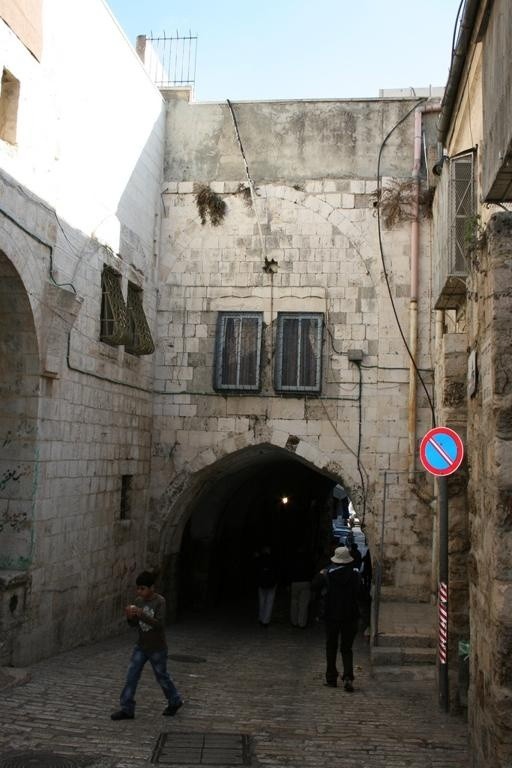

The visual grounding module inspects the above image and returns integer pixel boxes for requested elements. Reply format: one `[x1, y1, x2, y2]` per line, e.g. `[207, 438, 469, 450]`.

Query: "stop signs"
[419, 427, 464, 477]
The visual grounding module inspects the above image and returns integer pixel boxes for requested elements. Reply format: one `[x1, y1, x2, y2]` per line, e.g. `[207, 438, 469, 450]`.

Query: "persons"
[258, 543, 372, 630]
[111, 572, 182, 720]
[310, 546, 371, 692]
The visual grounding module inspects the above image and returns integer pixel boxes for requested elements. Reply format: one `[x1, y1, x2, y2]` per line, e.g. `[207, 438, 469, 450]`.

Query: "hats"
[331, 547, 354, 564]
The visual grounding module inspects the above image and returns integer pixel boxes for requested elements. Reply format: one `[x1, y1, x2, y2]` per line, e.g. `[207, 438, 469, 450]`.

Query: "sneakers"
[162, 700, 184, 717]
[343, 678, 353, 690]
[110, 711, 134, 720]
[323, 680, 336, 687]
